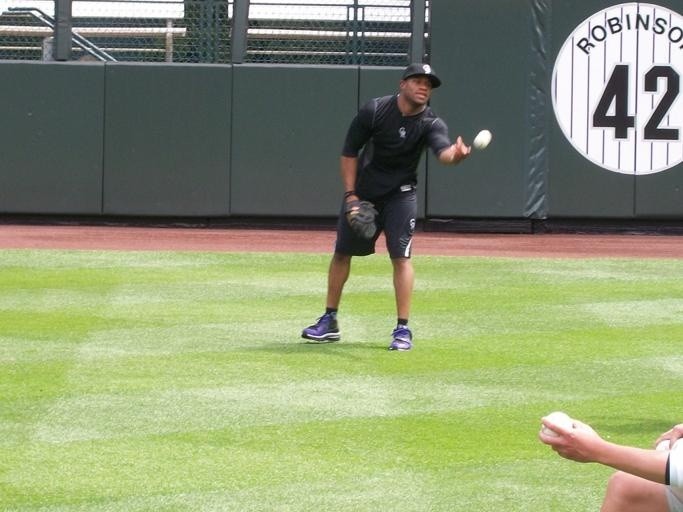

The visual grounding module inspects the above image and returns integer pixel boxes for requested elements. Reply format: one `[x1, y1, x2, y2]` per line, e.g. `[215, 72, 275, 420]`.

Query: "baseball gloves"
[344, 199, 380, 239]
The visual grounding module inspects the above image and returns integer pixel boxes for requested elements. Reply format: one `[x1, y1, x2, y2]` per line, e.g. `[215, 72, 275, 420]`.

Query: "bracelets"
[344, 191, 360, 200]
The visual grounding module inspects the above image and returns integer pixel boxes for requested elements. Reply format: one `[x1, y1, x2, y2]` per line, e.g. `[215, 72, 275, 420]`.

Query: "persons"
[298, 61, 471, 352]
[537, 410, 682, 512]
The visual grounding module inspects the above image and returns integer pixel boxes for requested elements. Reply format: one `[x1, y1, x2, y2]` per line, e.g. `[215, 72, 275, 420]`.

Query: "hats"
[403, 63, 441, 89]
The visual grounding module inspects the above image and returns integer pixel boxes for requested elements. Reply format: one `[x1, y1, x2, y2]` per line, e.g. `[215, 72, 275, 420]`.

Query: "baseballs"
[473, 129, 493, 149]
[541, 412, 572, 437]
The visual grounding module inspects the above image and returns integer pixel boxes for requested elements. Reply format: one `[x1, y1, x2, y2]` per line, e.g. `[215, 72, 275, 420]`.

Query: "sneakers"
[391, 324, 412, 351]
[300, 313, 339, 341]
